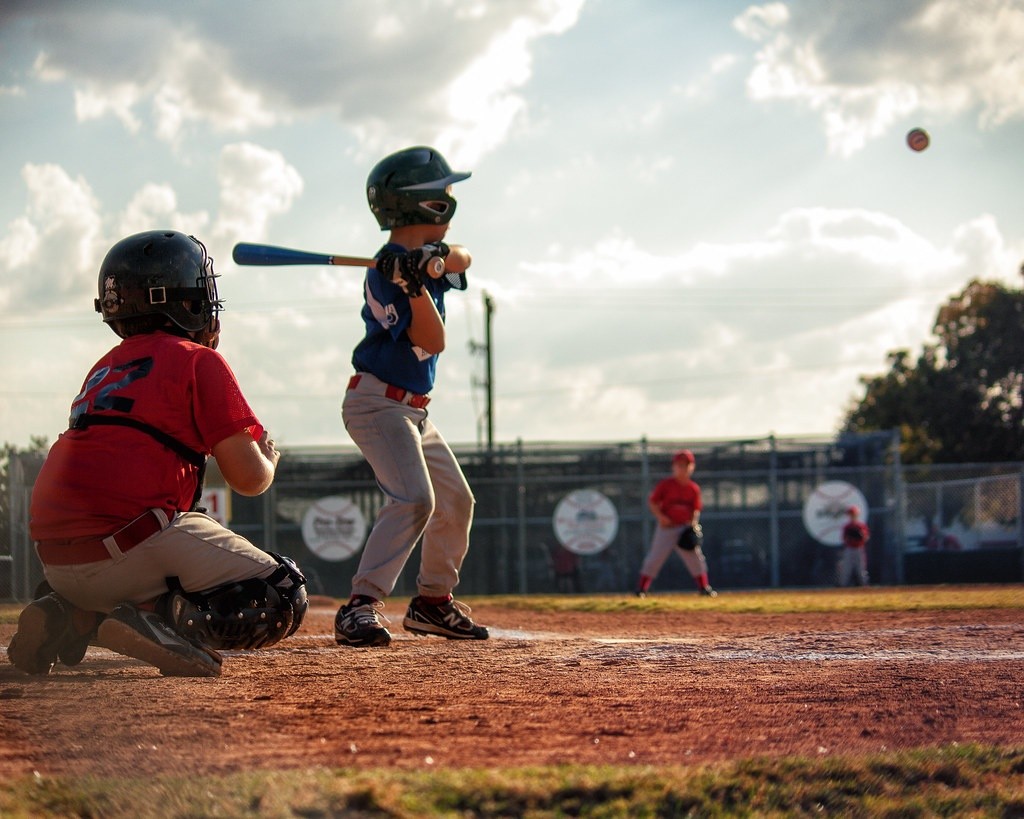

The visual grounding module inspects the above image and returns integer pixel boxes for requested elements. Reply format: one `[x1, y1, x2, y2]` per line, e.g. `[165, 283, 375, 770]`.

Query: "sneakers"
[334, 601, 394, 646]
[6, 592, 70, 675]
[401, 596, 489, 640]
[98, 603, 222, 678]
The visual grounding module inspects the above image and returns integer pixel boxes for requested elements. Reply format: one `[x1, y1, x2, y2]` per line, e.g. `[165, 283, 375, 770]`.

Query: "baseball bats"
[232, 242, 447, 279]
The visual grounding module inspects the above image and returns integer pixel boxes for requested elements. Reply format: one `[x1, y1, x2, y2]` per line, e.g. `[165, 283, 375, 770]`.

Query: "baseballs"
[906, 128, 931, 152]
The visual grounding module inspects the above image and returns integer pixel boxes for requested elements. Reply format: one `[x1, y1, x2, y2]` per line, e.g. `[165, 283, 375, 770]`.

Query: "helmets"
[93, 231, 225, 338]
[366, 145, 471, 230]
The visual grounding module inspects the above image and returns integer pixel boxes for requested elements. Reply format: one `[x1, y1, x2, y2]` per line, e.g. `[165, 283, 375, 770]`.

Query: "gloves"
[377, 252, 426, 297]
[402, 241, 451, 274]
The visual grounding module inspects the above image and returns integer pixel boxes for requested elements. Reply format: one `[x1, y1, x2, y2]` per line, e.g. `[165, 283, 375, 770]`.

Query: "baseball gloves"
[843, 527, 864, 540]
[677, 525, 704, 551]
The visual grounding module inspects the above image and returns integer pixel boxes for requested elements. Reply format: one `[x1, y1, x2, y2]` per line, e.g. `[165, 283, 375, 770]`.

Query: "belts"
[346, 376, 431, 408]
[38, 508, 175, 565]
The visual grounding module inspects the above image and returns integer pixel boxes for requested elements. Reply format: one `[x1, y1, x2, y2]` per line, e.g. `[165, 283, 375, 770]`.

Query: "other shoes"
[700, 585, 718, 597]
[636, 591, 646, 598]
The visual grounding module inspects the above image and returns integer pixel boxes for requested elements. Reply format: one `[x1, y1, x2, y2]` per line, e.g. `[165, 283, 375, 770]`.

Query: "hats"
[672, 450, 695, 463]
[848, 507, 860, 515]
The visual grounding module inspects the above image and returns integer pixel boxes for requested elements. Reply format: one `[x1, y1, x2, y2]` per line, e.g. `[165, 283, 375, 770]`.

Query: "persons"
[8, 230, 309, 679]
[636, 449, 715, 598]
[837, 506, 870, 587]
[335, 145, 490, 646]
